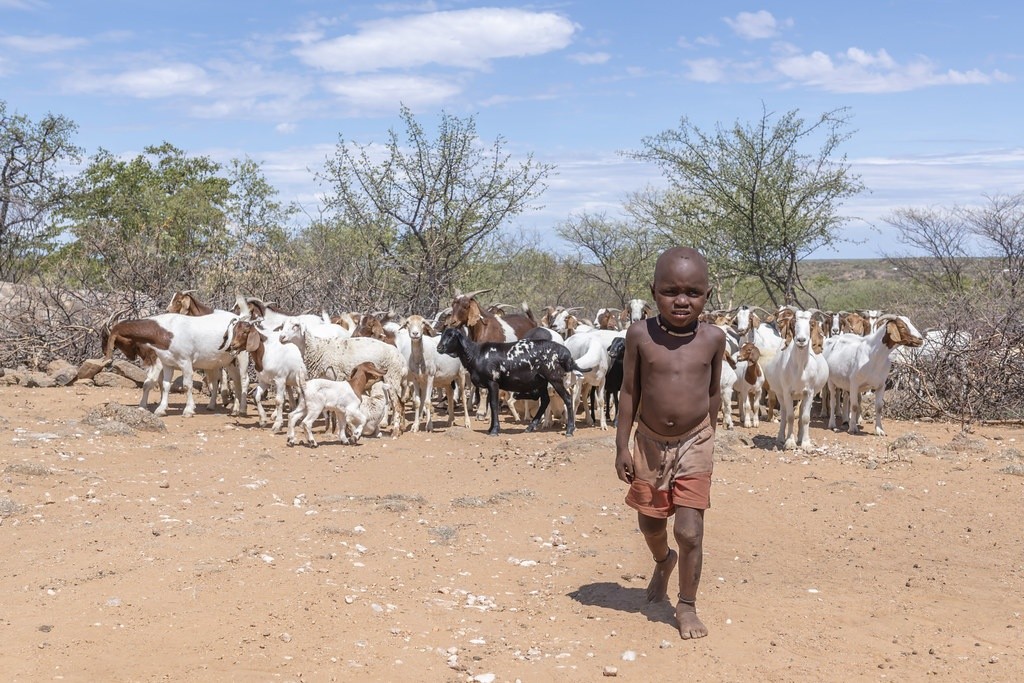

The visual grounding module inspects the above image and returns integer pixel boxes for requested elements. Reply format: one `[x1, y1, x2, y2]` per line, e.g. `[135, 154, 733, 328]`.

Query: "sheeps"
[96, 276, 975, 455]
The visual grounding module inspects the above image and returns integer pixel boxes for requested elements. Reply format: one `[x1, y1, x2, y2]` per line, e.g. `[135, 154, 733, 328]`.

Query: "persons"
[615, 246, 727, 640]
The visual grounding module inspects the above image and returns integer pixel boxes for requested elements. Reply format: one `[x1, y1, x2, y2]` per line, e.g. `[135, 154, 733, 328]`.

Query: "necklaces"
[656, 314, 700, 337]
